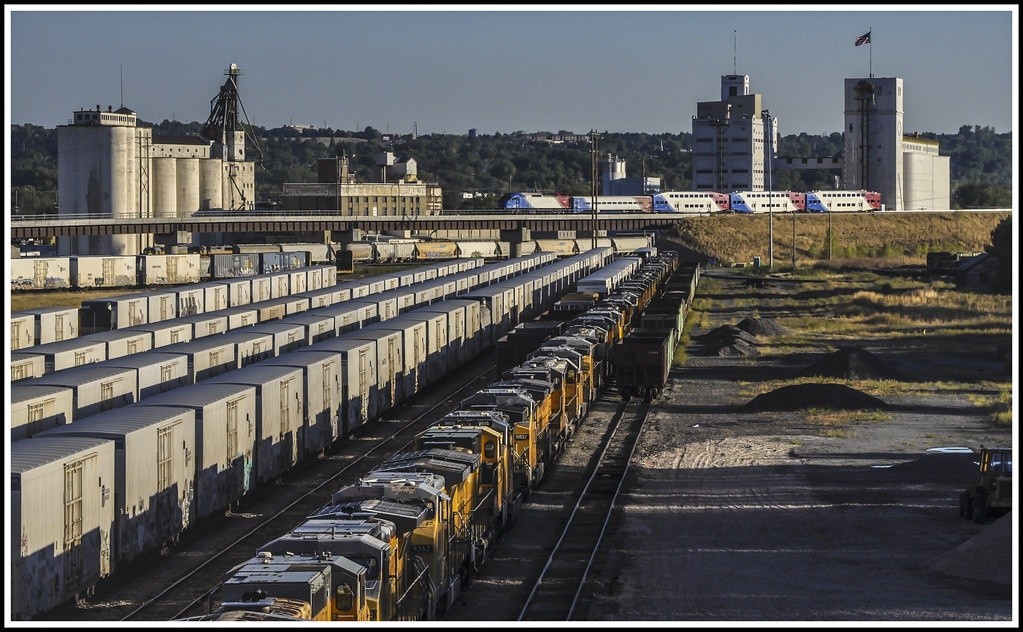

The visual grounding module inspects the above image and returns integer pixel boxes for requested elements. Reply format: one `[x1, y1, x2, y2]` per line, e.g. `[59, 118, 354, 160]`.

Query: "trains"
[497, 188, 882, 214]
[208, 246, 679, 622]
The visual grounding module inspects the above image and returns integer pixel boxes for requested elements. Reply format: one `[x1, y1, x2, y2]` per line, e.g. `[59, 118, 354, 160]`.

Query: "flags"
[855, 31, 870, 47]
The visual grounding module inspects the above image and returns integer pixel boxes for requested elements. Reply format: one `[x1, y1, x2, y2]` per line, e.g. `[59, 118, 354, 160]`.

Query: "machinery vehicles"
[960, 444, 1012, 524]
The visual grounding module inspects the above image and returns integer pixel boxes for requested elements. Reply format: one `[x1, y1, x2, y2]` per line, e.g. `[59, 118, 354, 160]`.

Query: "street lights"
[761, 110, 774, 269]
